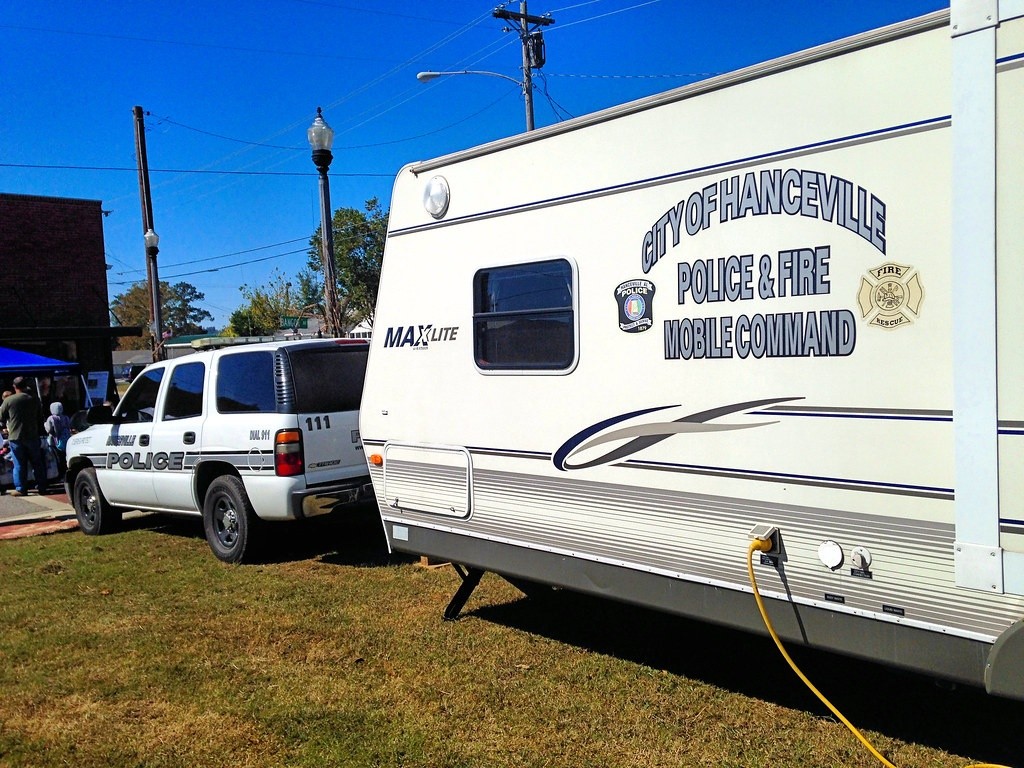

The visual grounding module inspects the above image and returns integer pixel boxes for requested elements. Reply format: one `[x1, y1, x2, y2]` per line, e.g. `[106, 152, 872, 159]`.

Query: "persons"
[0, 376, 54, 496]
[44, 402, 71, 482]
[86, 394, 120, 425]
[0, 391, 12, 404]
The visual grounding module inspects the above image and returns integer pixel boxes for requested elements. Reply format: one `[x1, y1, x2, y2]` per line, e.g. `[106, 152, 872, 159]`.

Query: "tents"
[1, 346, 96, 487]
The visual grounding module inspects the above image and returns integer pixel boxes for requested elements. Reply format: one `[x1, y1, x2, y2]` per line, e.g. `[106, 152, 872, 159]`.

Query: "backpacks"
[51, 416, 71, 451]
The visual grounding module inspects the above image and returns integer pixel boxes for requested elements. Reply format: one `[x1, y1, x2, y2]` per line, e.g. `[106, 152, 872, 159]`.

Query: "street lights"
[143, 227, 167, 361]
[415, 68, 536, 132]
[307, 108, 338, 334]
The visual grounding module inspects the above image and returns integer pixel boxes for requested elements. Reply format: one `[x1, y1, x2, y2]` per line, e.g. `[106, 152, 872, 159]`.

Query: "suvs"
[121, 365, 143, 382]
[63, 337, 374, 565]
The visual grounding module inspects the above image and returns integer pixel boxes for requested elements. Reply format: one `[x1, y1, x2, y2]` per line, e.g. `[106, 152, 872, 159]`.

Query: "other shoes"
[11, 490, 26, 496]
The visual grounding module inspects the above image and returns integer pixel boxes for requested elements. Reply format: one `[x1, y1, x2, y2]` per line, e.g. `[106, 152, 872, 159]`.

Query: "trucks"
[359, 4, 1024, 716]
